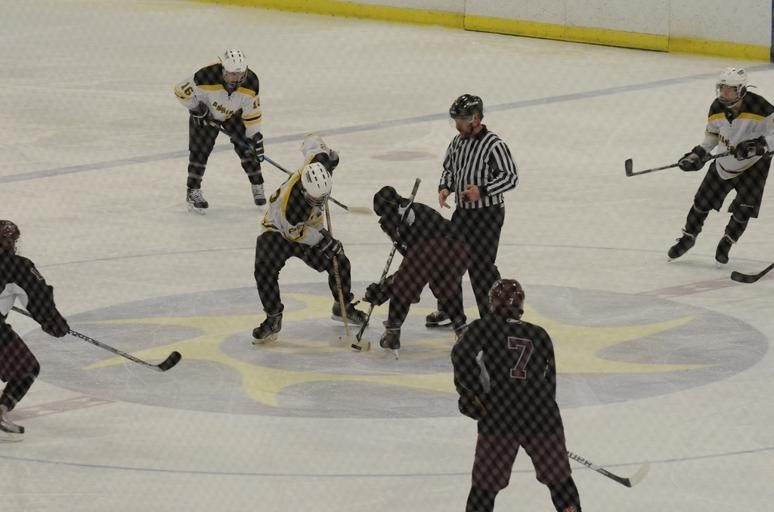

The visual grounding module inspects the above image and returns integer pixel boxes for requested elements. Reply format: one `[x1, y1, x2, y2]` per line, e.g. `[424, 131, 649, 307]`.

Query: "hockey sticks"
[624, 151, 732, 176]
[354, 179, 422, 350]
[10, 306, 181, 372]
[565, 452, 650, 487]
[209, 122, 373, 216]
[327, 201, 355, 348]
[730, 261, 774, 283]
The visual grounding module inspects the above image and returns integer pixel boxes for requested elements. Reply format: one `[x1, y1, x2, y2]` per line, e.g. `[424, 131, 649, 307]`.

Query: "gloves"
[458, 395, 482, 419]
[40, 315, 71, 338]
[190, 101, 213, 128]
[733, 134, 765, 161]
[364, 282, 389, 307]
[679, 145, 709, 171]
[245, 131, 265, 162]
[317, 228, 344, 261]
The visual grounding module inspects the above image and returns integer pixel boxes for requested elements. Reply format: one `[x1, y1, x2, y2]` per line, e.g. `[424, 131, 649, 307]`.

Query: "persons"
[421, 94, 521, 321]
[0, 220, 69, 434]
[249, 131, 369, 341]
[450, 277, 585, 512]
[361, 184, 473, 352]
[666, 68, 772, 263]
[173, 48, 269, 208]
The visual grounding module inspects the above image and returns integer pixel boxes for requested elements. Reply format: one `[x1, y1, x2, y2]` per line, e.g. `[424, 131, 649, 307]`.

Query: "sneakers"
[426, 309, 451, 324]
[251, 182, 267, 206]
[187, 186, 209, 208]
[715, 234, 736, 264]
[380, 327, 402, 349]
[668, 228, 696, 258]
[332, 299, 369, 323]
[0, 405, 26, 434]
[253, 312, 282, 339]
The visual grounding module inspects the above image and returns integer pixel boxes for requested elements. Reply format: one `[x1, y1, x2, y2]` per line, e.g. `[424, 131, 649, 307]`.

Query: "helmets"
[0, 220, 21, 240]
[450, 94, 484, 118]
[717, 67, 748, 98]
[488, 278, 524, 313]
[302, 162, 333, 198]
[221, 48, 249, 73]
